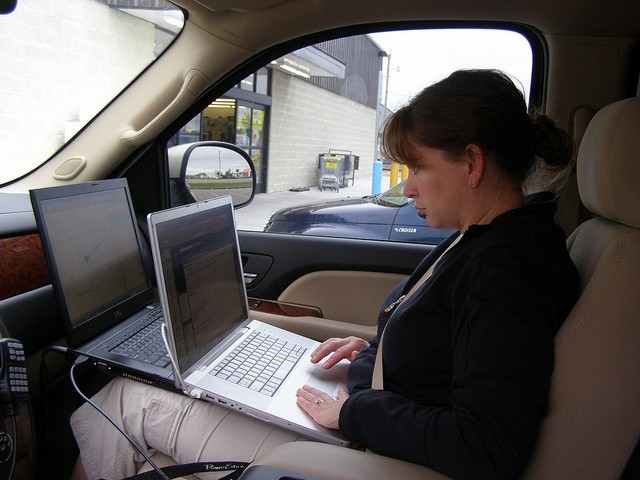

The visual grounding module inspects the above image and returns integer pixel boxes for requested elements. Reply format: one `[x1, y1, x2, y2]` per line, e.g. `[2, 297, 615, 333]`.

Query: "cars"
[260, 166, 458, 246]
[0, 0, 631, 480]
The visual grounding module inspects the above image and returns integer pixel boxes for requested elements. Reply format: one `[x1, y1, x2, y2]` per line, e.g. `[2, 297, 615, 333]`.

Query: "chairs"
[136, 97, 640, 480]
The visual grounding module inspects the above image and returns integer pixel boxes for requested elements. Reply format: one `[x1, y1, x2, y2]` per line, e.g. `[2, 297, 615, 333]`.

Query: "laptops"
[29, 177, 182, 393]
[147, 195, 351, 448]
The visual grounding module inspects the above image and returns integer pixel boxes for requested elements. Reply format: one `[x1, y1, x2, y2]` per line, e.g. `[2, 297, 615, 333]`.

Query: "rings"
[315, 399, 326, 406]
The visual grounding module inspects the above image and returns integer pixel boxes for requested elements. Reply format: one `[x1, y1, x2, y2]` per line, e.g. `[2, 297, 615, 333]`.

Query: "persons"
[69, 68, 586, 478]
